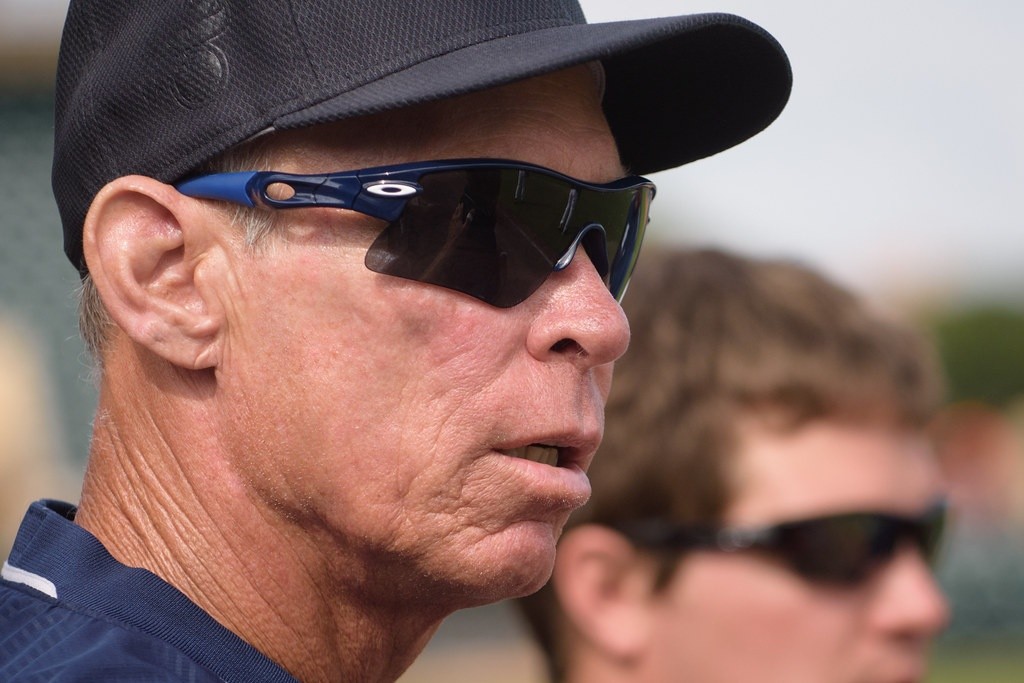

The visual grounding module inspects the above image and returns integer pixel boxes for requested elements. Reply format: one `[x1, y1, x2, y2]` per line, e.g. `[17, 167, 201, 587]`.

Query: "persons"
[505, 241, 952, 683]
[1, 1, 792, 683]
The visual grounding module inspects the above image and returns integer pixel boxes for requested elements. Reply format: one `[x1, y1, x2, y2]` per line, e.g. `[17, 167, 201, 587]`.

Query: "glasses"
[618, 498, 949, 587]
[174, 157, 657, 310]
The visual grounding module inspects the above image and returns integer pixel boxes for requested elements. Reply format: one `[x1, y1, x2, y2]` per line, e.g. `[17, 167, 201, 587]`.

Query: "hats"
[53, 1, 793, 281]
